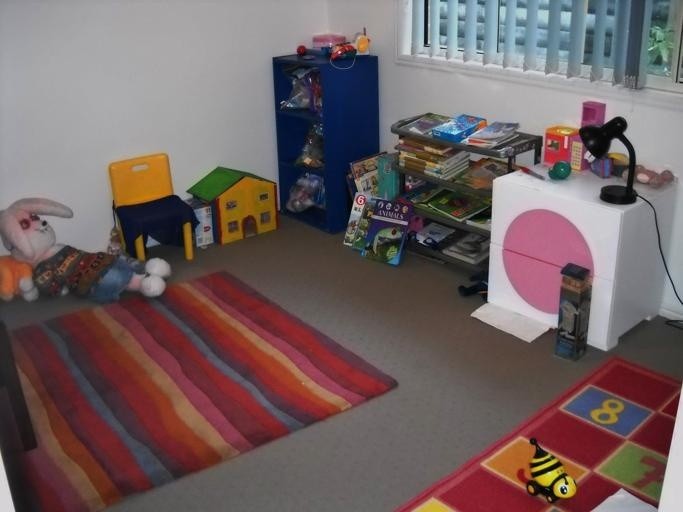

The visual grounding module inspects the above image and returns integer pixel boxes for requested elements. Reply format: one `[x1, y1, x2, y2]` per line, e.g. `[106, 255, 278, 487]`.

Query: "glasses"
[579, 116, 637, 204]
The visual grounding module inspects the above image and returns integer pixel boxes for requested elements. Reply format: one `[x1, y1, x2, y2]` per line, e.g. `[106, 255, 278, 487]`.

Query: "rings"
[519, 166, 545, 181]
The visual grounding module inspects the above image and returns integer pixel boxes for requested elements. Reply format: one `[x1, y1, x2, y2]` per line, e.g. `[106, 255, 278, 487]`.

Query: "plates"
[388, 113, 542, 281]
[486, 157, 678, 351]
[271, 54, 378, 232]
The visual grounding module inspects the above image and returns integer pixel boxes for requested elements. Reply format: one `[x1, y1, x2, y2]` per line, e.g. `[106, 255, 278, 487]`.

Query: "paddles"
[396, 358, 681, 512]
[10, 270, 398, 512]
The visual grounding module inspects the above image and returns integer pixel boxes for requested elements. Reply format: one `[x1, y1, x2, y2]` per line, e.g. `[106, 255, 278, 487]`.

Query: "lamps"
[109, 153, 194, 263]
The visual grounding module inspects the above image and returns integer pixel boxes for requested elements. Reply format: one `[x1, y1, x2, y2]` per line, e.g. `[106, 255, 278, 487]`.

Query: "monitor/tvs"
[343, 112, 520, 265]
